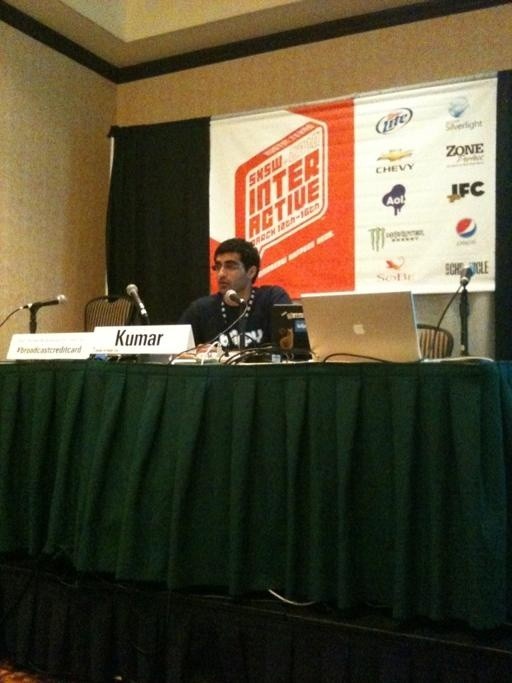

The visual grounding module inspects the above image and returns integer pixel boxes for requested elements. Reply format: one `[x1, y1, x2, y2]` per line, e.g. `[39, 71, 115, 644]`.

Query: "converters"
[242, 350, 273, 363]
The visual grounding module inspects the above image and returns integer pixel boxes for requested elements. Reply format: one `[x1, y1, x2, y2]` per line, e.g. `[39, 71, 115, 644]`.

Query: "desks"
[0, 357, 511, 629]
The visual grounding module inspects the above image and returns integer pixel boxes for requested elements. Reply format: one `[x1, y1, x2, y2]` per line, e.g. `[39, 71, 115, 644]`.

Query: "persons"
[178, 238, 293, 354]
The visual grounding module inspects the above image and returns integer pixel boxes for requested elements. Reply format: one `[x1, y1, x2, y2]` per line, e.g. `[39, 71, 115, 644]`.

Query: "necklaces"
[221, 289, 255, 324]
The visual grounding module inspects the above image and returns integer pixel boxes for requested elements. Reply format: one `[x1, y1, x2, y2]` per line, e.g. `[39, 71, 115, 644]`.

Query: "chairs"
[84, 295, 134, 332]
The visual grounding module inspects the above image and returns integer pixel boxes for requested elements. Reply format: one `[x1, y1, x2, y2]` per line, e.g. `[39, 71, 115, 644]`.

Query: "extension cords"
[196, 348, 270, 361]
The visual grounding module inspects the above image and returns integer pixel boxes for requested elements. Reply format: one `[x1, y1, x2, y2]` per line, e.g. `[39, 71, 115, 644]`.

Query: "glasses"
[211, 262, 248, 271]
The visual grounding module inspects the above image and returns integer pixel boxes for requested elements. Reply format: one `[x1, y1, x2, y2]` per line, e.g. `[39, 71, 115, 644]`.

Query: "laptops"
[270, 303, 312, 361]
[301, 290, 423, 362]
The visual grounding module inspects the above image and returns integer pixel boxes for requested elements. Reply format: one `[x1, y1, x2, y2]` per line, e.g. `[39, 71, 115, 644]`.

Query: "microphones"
[21, 294, 67, 311]
[126, 285, 149, 318]
[460, 262, 476, 285]
[225, 289, 245, 306]
